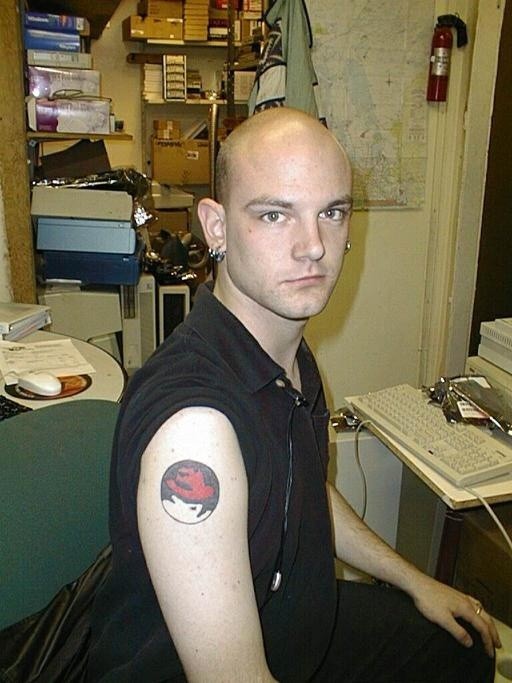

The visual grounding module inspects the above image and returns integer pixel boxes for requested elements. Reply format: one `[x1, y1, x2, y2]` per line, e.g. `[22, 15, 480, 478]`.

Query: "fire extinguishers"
[427, 14, 468, 101]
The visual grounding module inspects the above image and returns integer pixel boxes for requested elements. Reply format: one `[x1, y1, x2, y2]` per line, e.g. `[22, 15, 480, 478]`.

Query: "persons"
[104, 103, 502, 682]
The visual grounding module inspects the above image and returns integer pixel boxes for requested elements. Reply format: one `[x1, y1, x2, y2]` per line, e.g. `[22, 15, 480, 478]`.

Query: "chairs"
[0, 398, 131, 628]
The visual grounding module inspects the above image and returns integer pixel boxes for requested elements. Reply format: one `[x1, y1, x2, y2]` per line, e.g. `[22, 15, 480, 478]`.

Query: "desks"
[2, 328, 127, 412]
[347, 375, 512, 511]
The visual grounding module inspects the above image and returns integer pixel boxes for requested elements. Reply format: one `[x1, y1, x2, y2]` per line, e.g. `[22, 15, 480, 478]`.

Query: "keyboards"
[0, 395, 32, 424]
[351, 383, 512, 489]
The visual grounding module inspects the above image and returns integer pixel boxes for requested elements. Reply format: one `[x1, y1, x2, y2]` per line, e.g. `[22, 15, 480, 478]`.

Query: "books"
[179, 1, 264, 43]
[182, 119, 207, 140]
[1, 298, 53, 341]
[140, 61, 165, 103]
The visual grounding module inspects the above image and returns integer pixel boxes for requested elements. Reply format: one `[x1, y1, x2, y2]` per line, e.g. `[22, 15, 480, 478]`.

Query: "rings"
[474, 606, 482, 615]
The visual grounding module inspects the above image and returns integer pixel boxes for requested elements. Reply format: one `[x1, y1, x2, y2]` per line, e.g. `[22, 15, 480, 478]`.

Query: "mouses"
[18, 372, 62, 396]
